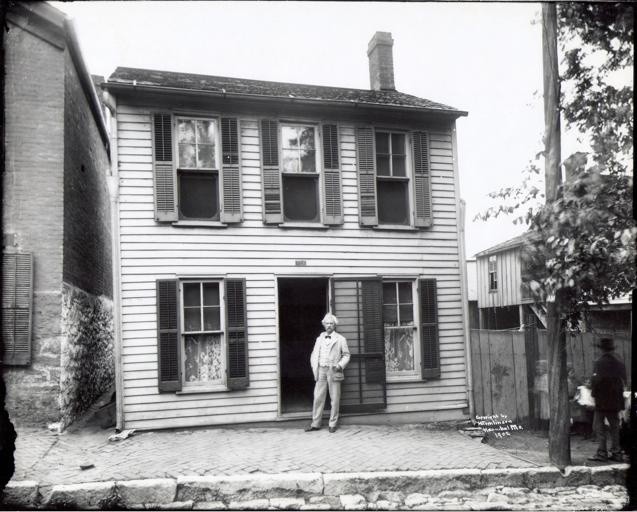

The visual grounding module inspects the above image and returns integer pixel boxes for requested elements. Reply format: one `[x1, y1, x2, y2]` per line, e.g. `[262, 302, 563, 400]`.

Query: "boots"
[609, 453, 622, 462]
[587, 450, 608, 462]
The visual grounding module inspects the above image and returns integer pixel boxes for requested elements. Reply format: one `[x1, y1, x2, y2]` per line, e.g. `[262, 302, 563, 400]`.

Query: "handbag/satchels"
[333, 372, 344, 381]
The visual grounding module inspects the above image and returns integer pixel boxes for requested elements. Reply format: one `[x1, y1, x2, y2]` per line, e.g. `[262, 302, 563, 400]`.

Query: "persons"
[585, 335, 628, 464]
[532, 359, 550, 439]
[566, 364, 581, 434]
[569, 375, 595, 441]
[304, 313, 351, 434]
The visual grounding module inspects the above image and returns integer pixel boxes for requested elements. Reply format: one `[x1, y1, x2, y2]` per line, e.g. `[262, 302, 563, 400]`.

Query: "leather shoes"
[305, 427, 320, 431]
[329, 427, 336, 433]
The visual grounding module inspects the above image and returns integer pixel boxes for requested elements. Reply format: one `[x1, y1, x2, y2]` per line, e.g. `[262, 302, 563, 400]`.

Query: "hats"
[594, 338, 615, 350]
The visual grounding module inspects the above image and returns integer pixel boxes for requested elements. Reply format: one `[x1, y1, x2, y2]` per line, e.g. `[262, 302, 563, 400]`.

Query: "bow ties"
[325, 336, 331, 339]
